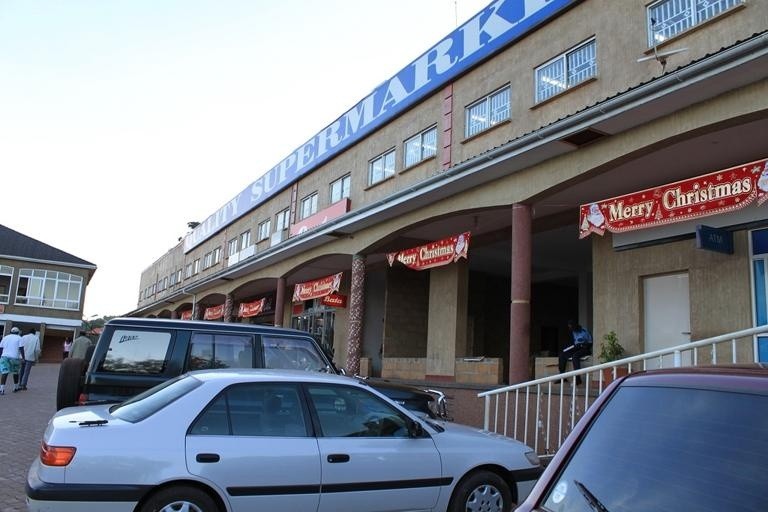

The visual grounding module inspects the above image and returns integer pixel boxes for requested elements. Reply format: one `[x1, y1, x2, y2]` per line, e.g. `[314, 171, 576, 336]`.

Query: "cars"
[25, 369, 543, 512]
[511, 362, 766, 512]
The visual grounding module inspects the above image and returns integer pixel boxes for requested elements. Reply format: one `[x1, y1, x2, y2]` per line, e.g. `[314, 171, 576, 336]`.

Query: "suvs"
[56, 317, 452, 423]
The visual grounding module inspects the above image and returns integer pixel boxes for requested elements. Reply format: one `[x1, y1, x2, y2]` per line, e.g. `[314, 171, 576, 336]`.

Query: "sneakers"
[13, 386, 27, 392]
[0, 390, 4, 395]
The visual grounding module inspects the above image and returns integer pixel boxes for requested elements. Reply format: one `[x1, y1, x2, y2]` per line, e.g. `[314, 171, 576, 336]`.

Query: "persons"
[18, 328, 41, 390]
[63, 336, 72, 360]
[0, 327, 25, 394]
[554, 320, 592, 385]
[69, 329, 92, 358]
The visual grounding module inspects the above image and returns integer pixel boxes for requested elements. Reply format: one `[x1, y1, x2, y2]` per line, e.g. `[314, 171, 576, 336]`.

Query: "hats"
[11, 327, 19, 333]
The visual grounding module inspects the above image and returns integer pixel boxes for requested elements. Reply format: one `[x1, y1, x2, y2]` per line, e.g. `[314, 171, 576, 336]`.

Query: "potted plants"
[598, 331, 632, 388]
